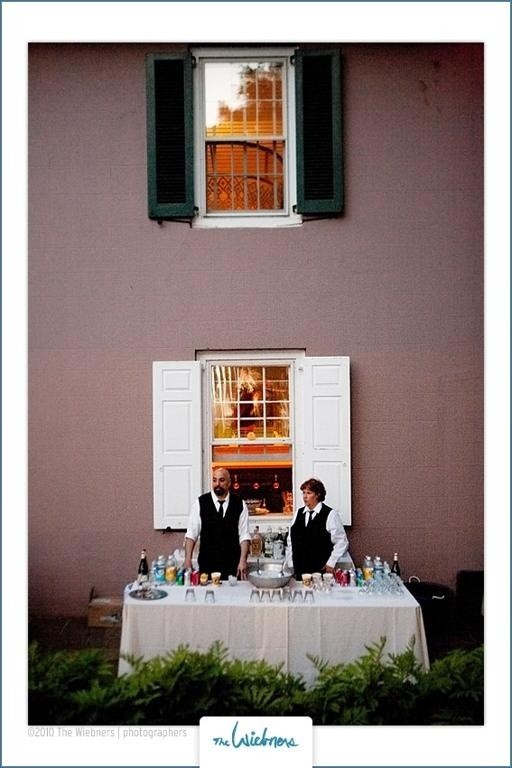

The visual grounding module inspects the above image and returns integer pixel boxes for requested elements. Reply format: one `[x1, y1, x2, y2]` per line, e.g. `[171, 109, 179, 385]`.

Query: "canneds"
[335, 568, 383, 588]
[191, 572, 200, 586]
[176, 571, 184, 586]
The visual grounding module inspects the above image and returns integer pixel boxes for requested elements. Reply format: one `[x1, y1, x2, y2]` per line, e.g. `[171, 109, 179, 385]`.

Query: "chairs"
[456, 568, 483, 613]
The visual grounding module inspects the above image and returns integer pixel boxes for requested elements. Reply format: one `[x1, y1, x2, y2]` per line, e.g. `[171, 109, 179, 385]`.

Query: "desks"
[115, 577, 427, 687]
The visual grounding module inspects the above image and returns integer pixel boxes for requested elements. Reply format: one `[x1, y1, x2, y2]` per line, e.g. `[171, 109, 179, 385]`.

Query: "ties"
[307, 510, 314, 527]
[218, 500, 225, 518]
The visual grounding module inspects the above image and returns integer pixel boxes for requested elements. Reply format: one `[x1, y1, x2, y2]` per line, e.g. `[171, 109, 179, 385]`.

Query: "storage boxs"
[88, 601, 125, 629]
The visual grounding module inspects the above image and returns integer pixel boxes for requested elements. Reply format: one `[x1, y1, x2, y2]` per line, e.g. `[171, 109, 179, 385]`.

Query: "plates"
[128, 588, 168, 600]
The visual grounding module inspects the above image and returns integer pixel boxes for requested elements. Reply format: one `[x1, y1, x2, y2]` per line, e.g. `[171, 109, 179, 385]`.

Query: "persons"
[282, 478, 349, 582]
[181, 468, 251, 582]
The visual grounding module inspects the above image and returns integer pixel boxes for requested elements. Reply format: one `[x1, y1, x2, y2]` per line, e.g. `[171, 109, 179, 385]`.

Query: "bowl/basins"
[244, 498, 261, 512]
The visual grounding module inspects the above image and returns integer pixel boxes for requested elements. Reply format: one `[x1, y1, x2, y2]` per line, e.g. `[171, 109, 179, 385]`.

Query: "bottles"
[362, 552, 401, 580]
[335, 567, 363, 586]
[138, 549, 149, 584]
[250, 524, 290, 559]
[152, 554, 209, 586]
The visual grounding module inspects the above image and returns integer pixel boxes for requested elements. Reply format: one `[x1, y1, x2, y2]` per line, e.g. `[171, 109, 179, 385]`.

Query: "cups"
[302, 571, 334, 598]
[228, 574, 237, 585]
[185, 588, 196, 602]
[137, 568, 161, 597]
[211, 571, 222, 585]
[248, 588, 314, 604]
[330, 586, 355, 600]
[283, 490, 292, 513]
[359, 571, 405, 603]
[204, 590, 216, 603]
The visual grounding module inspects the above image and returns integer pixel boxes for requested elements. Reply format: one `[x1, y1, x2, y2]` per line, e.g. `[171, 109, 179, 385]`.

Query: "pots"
[240, 564, 293, 588]
